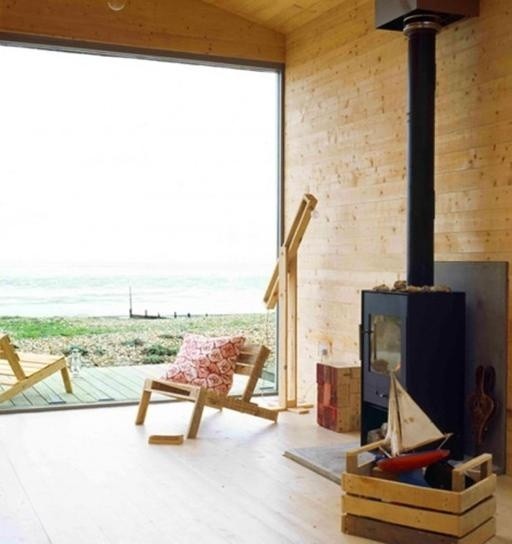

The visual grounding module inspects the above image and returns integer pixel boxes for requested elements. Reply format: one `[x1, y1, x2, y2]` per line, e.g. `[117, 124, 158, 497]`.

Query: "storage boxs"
[341, 440, 495, 544]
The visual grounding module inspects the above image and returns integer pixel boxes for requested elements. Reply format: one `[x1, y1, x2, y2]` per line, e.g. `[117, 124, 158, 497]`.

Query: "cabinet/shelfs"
[317, 361, 361, 433]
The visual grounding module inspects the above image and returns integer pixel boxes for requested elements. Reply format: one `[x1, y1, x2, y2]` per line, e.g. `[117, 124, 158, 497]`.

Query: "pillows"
[165, 332, 245, 395]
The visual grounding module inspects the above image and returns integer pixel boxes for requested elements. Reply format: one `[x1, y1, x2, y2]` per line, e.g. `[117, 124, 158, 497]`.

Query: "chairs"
[0, 331, 281, 437]
